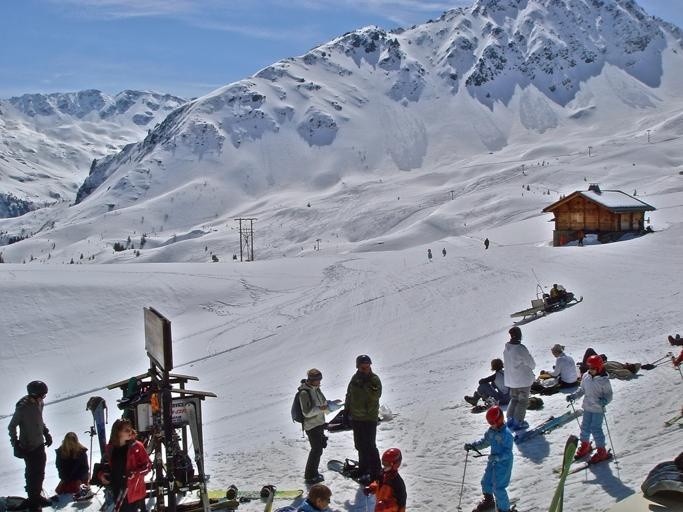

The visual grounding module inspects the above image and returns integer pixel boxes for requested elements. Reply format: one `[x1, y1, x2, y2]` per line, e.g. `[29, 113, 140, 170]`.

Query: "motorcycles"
[509, 281, 583, 325]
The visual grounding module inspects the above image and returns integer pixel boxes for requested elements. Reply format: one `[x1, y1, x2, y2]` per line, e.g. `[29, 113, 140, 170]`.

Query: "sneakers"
[476, 497, 493, 511]
[675, 334, 680, 339]
[513, 423, 528, 430]
[667, 336, 674, 346]
[506, 417, 513, 428]
[590, 448, 607, 463]
[464, 396, 477, 406]
[573, 442, 590, 458]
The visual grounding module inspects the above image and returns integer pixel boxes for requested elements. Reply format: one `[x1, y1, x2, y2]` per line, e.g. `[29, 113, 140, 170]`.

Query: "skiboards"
[665, 409, 683, 428]
[547, 436, 579, 512]
[470, 497, 539, 512]
[513, 409, 583, 445]
[552, 444, 630, 478]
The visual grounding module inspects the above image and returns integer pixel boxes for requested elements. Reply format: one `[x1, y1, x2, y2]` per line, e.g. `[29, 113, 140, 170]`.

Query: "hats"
[308, 369, 321, 381]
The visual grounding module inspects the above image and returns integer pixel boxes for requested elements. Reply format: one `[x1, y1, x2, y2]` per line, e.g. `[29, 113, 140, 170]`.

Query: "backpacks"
[290, 389, 312, 439]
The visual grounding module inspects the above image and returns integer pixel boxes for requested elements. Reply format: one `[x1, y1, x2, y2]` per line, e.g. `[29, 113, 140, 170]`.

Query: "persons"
[484, 238, 490, 250]
[566, 355, 612, 464]
[292, 367, 342, 483]
[540, 344, 578, 388]
[464, 359, 510, 407]
[54, 430, 95, 501]
[503, 326, 536, 430]
[464, 406, 518, 512]
[8, 381, 54, 512]
[364, 447, 408, 512]
[550, 284, 563, 300]
[344, 354, 382, 482]
[293, 485, 332, 512]
[96, 418, 153, 512]
[578, 229, 584, 247]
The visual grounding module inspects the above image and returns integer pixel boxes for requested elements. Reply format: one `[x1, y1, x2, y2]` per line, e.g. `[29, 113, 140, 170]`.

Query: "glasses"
[381, 461, 389, 466]
[586, 367, 594, 370]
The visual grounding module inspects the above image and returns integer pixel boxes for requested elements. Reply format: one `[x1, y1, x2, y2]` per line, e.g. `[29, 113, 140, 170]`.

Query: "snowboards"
[196, 488, 303, 500]
[326, 460, 386, 487]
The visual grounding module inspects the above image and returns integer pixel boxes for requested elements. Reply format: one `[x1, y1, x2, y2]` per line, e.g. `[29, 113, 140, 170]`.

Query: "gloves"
[463, 444, 470, 450]
[363, 486, 371, 496]
[45, 434, 51, 447]
[598, 399, 607, 406]
[672, 358, 680, 366]
[487, 455, 499, 464]
[565, 395, 570, 402]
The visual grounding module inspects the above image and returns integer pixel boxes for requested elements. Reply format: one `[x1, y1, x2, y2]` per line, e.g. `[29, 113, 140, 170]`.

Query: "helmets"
[381, 449, 402, 468]
[356, 355, 371, 364]
[491, 358, 502, 370]
[585, 355, 603, 373]
[485, 406, 503, 427]
[27, 381, 47, 398]
